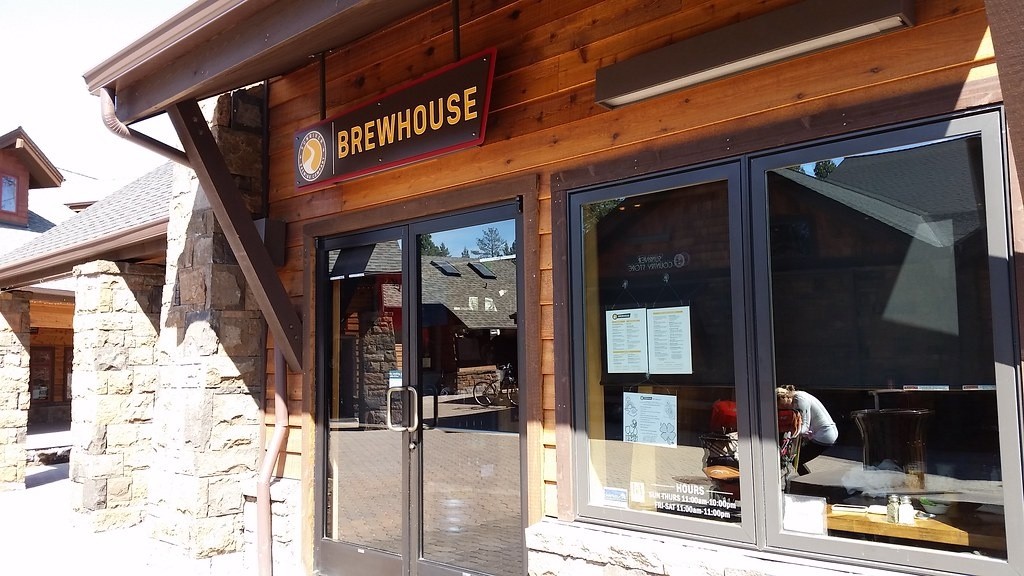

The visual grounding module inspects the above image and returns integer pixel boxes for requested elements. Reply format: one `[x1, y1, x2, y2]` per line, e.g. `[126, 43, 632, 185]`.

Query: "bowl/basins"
[921, 503, 952, 514]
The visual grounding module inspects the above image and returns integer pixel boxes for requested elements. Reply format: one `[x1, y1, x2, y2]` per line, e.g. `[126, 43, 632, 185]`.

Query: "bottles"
[898, 494, 914, 525]
[887, 494, 899, 524]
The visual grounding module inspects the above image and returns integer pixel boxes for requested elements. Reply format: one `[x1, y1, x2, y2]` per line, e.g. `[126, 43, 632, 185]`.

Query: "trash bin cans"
[849, 408, 930, 473]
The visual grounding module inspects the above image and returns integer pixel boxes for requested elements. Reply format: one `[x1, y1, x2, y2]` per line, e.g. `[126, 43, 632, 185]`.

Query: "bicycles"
[472, 363, 519, 408]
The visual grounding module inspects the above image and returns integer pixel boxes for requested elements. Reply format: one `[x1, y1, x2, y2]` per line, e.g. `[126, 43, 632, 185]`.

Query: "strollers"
[778, 409, 802, 474]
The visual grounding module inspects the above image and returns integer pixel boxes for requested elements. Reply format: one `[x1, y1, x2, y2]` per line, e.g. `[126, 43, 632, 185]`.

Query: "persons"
[777, 386, 838, 477]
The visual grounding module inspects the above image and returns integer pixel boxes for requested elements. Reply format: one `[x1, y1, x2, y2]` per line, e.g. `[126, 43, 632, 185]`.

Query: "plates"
[867, 505, 887, 515]
